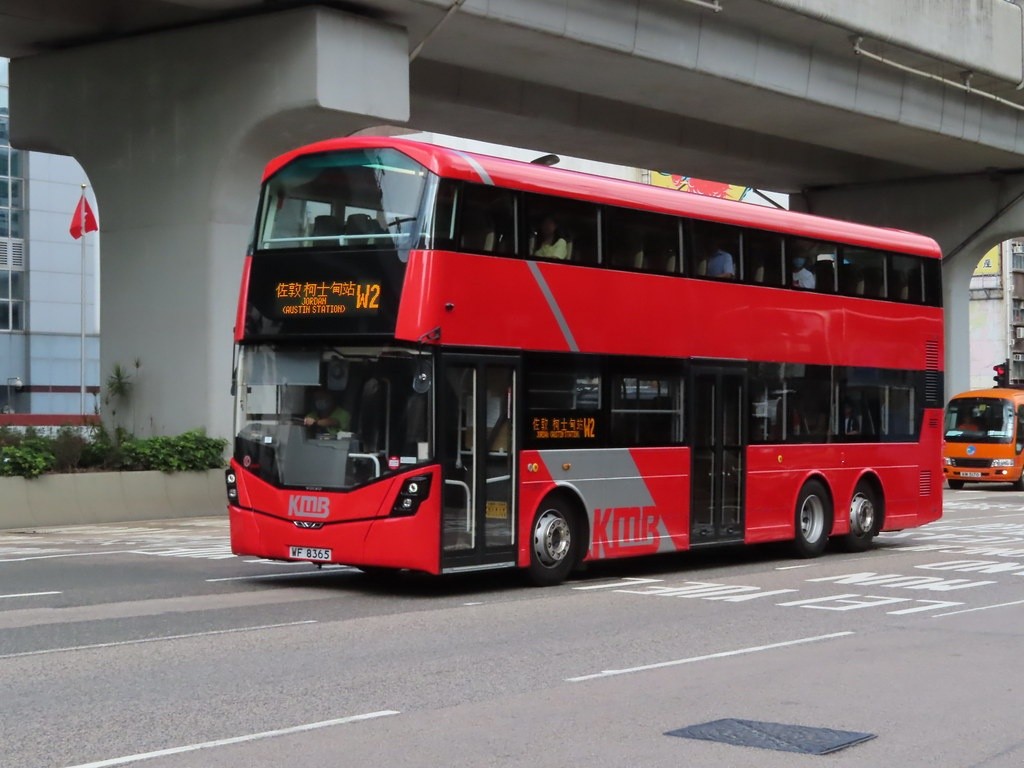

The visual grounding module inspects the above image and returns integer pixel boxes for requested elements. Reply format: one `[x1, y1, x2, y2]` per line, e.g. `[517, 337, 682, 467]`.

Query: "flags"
[69, 196, 98, 239]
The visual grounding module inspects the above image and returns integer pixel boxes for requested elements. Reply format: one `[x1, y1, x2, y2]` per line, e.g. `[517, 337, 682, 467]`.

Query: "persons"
[960, 415, 977, 431]
[304, 391, 351, 435]
[534, 215, 568, 258]
[786, 251, 815, 288]
[701, 239, 733, 279]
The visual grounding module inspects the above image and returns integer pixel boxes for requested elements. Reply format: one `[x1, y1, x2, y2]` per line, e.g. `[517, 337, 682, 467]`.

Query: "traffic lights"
[992, 364, 1008, 391]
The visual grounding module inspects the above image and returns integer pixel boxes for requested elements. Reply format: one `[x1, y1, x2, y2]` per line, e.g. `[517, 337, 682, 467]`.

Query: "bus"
[224, 134, 946, 590]
[945, 389, 1024, 490]
